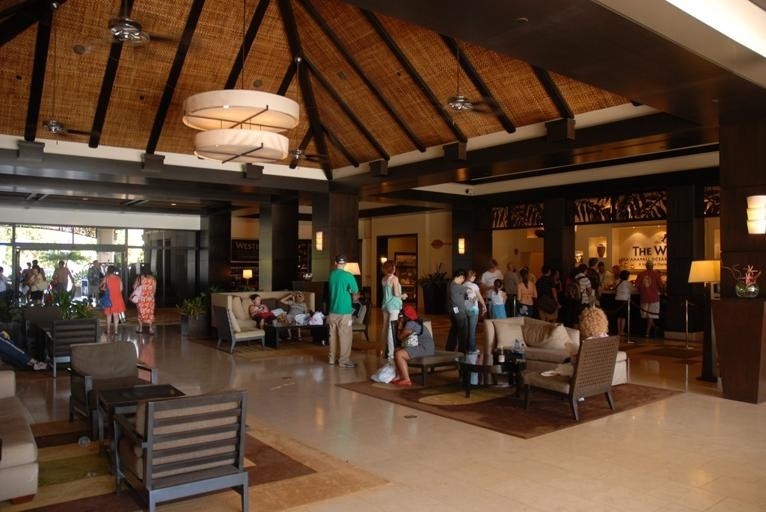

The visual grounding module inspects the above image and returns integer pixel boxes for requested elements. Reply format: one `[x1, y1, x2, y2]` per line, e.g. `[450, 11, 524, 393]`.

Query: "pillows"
[492, 321, 572, 350]
[225, 294, 259, 331]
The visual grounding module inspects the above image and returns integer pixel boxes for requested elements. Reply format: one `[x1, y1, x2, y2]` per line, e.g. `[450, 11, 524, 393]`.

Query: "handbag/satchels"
[128, 285, 142, 303]
[401, 332, 418, 348]
[255, 312, 274, 319]
[97, 288, 112, 309]
[540, 295, 556, 314]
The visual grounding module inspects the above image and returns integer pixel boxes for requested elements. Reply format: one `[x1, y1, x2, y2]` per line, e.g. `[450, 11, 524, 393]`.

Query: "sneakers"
[339, 360, 359, 369]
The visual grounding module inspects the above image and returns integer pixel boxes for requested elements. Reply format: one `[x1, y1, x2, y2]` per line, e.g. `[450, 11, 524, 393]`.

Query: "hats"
[335, 254, 348, 264]
[402, 303, 418, 321]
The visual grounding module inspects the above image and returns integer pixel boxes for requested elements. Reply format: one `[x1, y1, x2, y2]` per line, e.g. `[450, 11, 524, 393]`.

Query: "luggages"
[44, 289, 52, 306]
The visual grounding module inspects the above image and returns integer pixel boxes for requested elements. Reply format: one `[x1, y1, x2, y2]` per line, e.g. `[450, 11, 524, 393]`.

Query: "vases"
[735, 282, 758, 297]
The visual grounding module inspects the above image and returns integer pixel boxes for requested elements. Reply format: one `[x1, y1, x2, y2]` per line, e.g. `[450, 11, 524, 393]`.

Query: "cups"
[498, 355, 505, 362]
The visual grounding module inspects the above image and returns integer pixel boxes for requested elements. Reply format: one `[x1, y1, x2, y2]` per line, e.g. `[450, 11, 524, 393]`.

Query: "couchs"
[208, 291, 314, 354]
[485, 316, 627, 402]
[0, 370, 41, 500]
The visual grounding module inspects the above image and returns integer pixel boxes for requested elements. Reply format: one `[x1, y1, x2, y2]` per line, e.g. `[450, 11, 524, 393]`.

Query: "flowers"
[726, 263, 762, 286]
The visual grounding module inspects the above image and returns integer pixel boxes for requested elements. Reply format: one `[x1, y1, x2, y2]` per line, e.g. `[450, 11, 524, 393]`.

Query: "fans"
[421, 35, 498, 114]
[286, 62, 330, 165]
[23, 11, 100, 139]
[71, 1, 200, 67]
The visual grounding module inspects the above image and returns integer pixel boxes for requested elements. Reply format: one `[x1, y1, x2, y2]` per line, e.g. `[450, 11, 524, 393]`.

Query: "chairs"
[388, 319, 461, 383]
[67, 340, 147, 437]
[521, 335, 621, 422]
[45, 318, 100, 378]
[336, 302, 372, 341]
[213, 304, 265, 353]
[22, 307, 61, 356]
[105, 390, 250, 510]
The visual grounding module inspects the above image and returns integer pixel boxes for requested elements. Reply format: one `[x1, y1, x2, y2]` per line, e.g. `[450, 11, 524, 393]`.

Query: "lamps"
[688, 259, 721, 384]
[242, 270, 254, 291]
[181, 0, 299, 161]
[745, 195, 766, 235]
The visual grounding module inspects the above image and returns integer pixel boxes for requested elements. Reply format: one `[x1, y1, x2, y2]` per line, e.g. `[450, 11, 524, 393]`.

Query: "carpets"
[94, 307, 180, 327]
[0, 413, 391, 512]
[335, 380, 686, 439]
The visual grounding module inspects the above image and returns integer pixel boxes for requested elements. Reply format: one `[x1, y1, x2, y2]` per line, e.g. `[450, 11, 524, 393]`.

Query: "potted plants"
[176, 287, 221, 340]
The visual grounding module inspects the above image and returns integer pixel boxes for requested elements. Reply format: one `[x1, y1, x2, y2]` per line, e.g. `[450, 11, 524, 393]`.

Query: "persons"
[279, 290, 307, 342]
[86, 260, 136, 335]
[381, 260, 408, 361]
[131, 263, 157, 334]
[392, 304, 435, 386]
[352, 293, 362, 318]
[321, 255, 360, 367]
[0, 259, 74, 305]
[446, 257, 664, 362]
[249, 294, 283, 344]
[0, 330, 48, 370]
